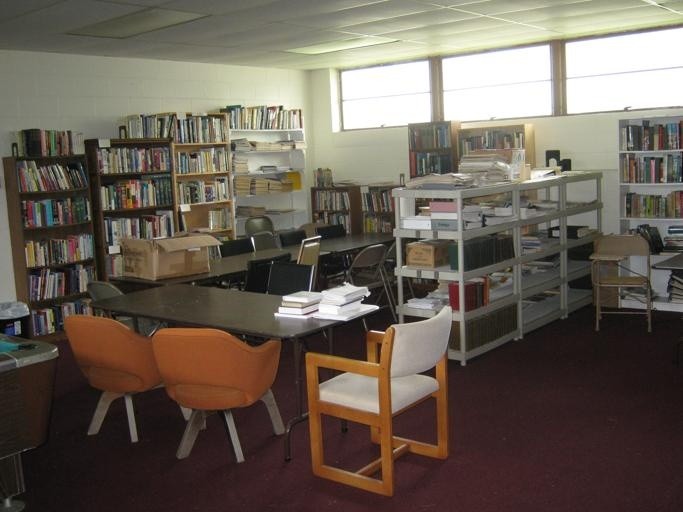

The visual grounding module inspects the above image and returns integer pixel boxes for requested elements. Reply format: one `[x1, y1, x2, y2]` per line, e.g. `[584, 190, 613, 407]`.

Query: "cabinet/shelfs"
[83, 138, 173, 281]
[360, 182, 404, 236]
[407, 121, 460, 179]
[228, 129, 311, 238]
[617, 116, 683, 313]
[2, 154, 104, 342]
[455, 125, 535, 177]
[152, 112, 236, 240]
[308, 182, 362, 234]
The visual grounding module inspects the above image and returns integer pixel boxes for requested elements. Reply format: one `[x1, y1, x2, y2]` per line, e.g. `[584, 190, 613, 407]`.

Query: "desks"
[0, 330, 61, 511]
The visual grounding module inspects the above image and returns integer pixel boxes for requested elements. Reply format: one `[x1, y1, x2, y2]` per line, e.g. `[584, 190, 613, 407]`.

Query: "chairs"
[591, 235, 651, 332]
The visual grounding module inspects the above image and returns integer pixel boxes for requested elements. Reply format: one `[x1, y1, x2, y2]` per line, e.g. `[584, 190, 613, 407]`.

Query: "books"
[18, 128, 103, 335]
[274, 285, 378, 321]
[220, 103, 304, 218]
[314, 168, 396, 232]
[100, 113, 175, 277]
[621, 118, 683, 301]
[402, 123, 599, 350]
[174, 118, 232, 241]
